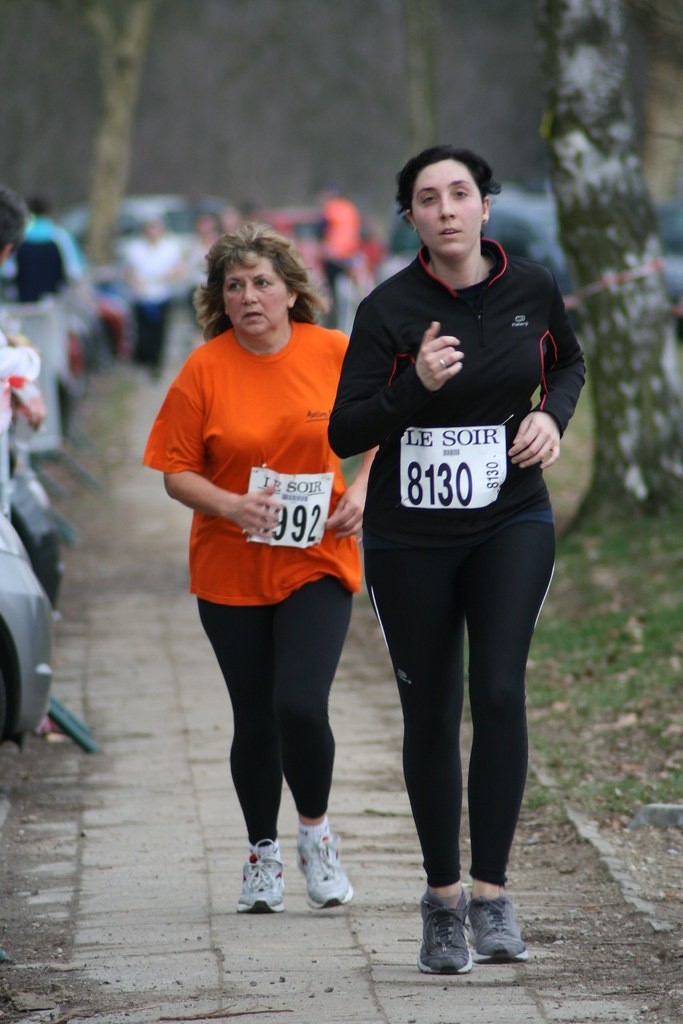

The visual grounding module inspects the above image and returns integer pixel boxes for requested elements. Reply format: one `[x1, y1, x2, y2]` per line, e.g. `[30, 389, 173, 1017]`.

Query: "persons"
[0, 182, 41, 382]
[140, 224, 379, 913]
[182, 207, 241, 327]
[327, 144, 587, 973]
[13, 195, 82, 302]
[312, 181, 361, 287]
[119, 218, 179, 366]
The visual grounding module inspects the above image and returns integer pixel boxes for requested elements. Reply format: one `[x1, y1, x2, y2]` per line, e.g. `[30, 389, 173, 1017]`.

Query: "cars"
[1, 196, 384, 757]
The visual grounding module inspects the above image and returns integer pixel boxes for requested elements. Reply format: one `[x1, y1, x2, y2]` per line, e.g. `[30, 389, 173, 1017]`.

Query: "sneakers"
[298, 830, 354, 909]
[467, 893, 528, 963]
[418, 888, 472, 974]
[236, 839, 285, 913]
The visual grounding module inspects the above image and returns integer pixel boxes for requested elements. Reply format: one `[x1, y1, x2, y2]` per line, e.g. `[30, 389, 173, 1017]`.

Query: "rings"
[440, 359, 445, 368]
